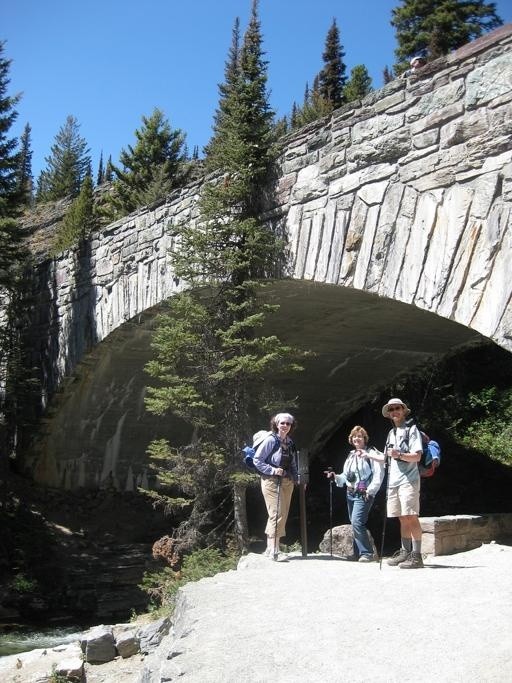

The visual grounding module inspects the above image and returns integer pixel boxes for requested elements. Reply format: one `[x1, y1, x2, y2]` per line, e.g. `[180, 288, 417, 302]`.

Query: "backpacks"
[243, 433, 281, 469]
[405, 423, 441, 479]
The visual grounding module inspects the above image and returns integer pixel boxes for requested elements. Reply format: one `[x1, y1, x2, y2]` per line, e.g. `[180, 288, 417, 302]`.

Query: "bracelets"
[398, 451, 402, 460]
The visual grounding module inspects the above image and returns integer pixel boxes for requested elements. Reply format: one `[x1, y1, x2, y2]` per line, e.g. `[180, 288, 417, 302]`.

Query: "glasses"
[279, 421, 291, 425]
[387, 406, 403, 411]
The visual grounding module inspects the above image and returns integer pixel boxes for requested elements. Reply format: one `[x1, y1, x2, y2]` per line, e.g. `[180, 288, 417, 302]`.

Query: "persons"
[355, 397, 426, 569]
[251, 411, 301, 563]
[323, 424, 386, 563]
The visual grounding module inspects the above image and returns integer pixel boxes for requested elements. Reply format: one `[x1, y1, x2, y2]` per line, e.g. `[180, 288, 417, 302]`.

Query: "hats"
[381, 396, 412, 418]
[251, 429, 272, 448]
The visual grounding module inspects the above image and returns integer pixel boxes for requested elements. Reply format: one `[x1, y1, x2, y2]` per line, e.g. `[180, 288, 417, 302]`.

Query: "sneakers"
[357, 553, 372, 562]
[264, 547, 290, 562]
[397, 550, 424, 569]
[386, 547, 411, 566]
